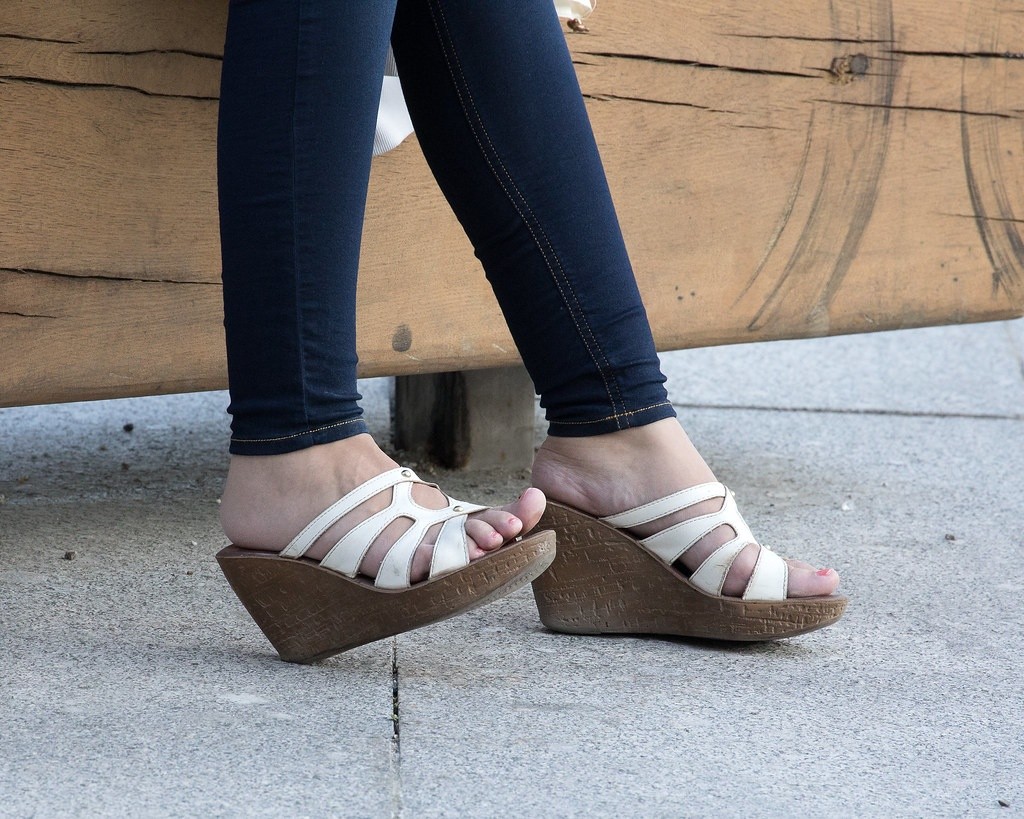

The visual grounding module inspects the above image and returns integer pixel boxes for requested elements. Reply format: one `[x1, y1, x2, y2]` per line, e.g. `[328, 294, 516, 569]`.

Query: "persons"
[215, 0, 846, 662]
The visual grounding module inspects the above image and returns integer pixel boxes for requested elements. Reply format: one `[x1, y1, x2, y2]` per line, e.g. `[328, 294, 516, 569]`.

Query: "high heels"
[522, 481, 846, 640]
[214, 464, 556, 665]
